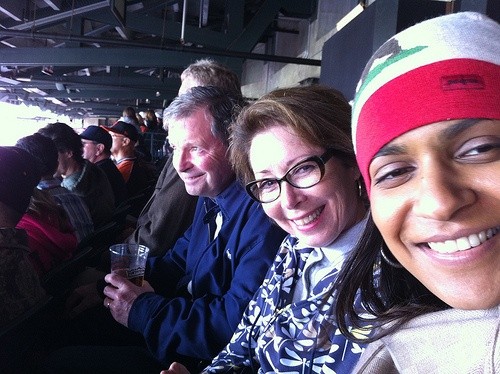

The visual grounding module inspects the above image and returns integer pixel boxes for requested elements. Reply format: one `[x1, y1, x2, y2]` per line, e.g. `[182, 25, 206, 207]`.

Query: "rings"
[108, 301, 112, 309]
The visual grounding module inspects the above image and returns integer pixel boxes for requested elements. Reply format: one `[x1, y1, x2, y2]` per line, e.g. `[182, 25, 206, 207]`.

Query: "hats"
[78, 125, 113, 148]
[100, 121, 138, 143]
[350, 12, 500, 197]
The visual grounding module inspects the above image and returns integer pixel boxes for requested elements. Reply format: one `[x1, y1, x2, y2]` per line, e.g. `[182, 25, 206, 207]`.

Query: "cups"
[109, 243, 150, 287]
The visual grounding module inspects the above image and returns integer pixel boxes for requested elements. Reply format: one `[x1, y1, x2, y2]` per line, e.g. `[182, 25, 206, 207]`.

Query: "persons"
[0, 58, 245, 374]
[103, 86, 286, 374]
[160, 86, 381, 374]
[318, 12, 500, 374]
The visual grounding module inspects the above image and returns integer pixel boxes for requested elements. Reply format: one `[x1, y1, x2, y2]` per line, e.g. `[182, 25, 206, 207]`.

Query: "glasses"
[245, 148, 335, 203]
